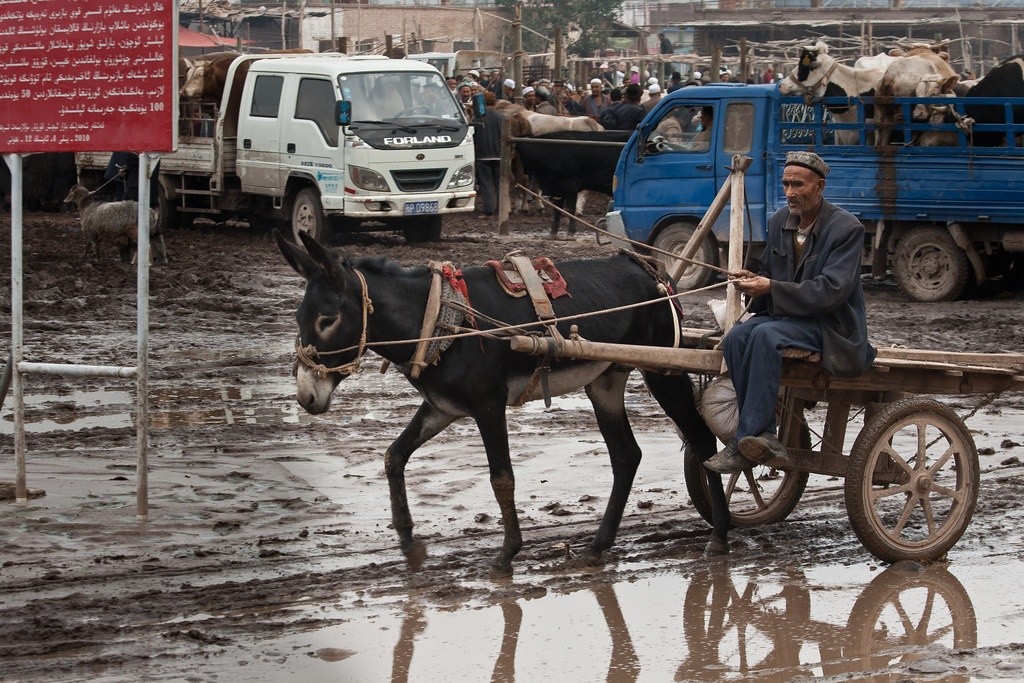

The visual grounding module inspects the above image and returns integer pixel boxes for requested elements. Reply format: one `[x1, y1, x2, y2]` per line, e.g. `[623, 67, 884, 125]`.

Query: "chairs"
[367, 78, 405, 120]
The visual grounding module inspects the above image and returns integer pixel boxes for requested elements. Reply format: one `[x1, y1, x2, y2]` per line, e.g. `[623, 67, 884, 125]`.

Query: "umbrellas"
[179, 24, 254, 48]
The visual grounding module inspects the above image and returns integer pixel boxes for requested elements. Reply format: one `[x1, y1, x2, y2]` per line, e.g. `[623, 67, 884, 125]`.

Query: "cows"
[177, 49, 312, 137]
[913, 54, 1024, 146]
[463, 98, 638, 240]
[778, 38, 958, 145]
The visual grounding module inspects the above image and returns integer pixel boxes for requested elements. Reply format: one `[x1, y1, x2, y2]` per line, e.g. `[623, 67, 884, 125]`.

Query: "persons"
[469, 92, 501, 217]
[411, 75, 464, 115]
[583, 78, 611, 121]
[458, 82, 473, 111]
[601, 83, 647, 130]
[640, 85, 663, 112]
[753, 68, 775, 84]
[523, 79, 588, 116]
[703, 150, 877, 473]
[590, 61, 659, 90]
[503, 79, 515, 103]
[649, 67, 742, 151]
[468, 68, 502, 99]
[658, 33, 673, 54]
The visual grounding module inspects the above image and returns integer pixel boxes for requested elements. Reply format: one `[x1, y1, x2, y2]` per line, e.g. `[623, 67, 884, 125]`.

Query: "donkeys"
[271, 227, 730, 578]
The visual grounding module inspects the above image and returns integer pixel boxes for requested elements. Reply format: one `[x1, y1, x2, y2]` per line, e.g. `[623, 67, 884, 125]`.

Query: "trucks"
[76, 50, 487, 250]
[593, 81, 1024, 304]
[401, 48, 549, 99]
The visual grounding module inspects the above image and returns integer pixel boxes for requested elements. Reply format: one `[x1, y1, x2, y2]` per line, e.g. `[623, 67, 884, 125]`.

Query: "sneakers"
[738, 431, 789, 469]
[703, 440, 760, 474]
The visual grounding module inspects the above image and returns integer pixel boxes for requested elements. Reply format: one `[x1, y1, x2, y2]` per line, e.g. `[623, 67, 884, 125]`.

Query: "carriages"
[271, 154, 1023, 570]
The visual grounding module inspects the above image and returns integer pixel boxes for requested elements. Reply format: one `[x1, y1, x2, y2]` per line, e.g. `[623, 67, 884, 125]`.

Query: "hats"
[504, 79, 515, 90]
[627, 83, 643, 100]
[539, 79, 551, 85]
[648, 77, 658, 85]
[694, 72, 702, 79]
[644, 71, 650, 78]
[469, 81, 479, 87]
[538, 85, 550, 97]
[610, 89, 622, 102]
[648, 83, 660, 93]
[478, 69, 487, 73]
[467, 70, 480, 78]
[671, 70, 683, 81]
[590, 78, 602, 84]
[462, 77, 473, 83]
[784, 151, 831, 180]
[599, 64, 608, 68]
[446, 77, 455, 81]
[489, 68, 499, 75]
[528, 78, 540, 86]
[457, 81, 470, 91]
[631, 66, 638, 72]
[522, 86, 534, 96]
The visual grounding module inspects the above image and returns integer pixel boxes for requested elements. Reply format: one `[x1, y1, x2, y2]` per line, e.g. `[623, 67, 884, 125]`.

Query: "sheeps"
[63, 185, 168, 267]
